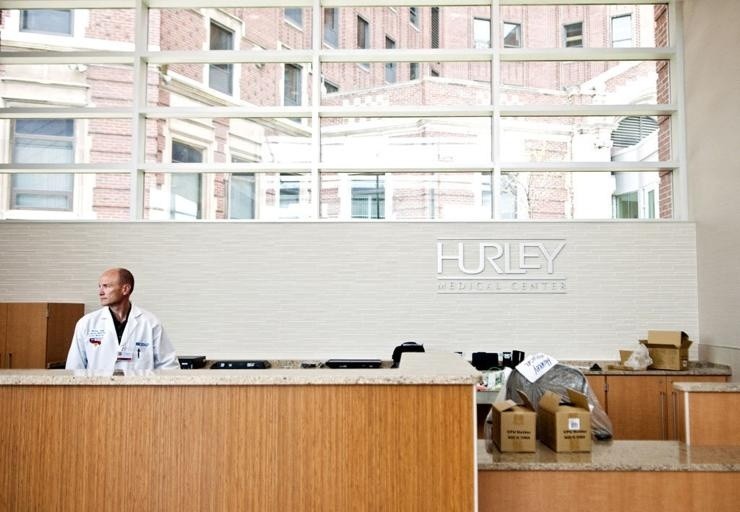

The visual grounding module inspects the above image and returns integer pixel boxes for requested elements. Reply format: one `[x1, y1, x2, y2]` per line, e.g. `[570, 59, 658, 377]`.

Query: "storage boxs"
[491, 389, 591, 455]
[636, 330, 694, 369]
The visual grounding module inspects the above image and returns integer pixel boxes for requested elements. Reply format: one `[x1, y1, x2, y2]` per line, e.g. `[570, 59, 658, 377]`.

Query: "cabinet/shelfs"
[2, 302, 85, 371]
[607, 375, 727, 439]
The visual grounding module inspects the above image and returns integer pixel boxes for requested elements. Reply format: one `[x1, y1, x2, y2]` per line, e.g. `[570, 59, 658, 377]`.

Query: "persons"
[64, 267, 179, 372]
